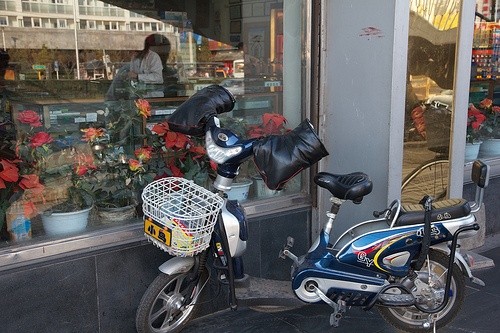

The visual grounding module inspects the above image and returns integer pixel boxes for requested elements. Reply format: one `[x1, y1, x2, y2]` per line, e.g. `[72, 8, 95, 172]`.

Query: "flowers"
[0, 97, 218, 209]
[251, 112, 293, 174]
[468, 98, 500, 141]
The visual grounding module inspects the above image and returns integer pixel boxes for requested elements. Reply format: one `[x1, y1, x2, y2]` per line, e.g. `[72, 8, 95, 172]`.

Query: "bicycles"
[401, 143, 450, 204]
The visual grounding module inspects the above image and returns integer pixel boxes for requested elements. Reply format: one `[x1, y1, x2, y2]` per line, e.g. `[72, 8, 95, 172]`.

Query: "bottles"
[472, 55, 500, 80]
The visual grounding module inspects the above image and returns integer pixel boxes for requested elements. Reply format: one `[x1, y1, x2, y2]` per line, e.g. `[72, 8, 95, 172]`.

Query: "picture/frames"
[229, 4, 242, 19]
[245, 20, 270, 60]
[229, 0, 242, 4]
[230, 20, 241, 34]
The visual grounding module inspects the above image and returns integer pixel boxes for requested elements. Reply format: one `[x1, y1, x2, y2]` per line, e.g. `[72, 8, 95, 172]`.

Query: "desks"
[5, 91, 277, 132]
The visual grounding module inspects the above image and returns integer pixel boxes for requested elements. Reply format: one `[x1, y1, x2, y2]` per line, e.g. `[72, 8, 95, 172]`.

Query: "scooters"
[133, 84, 490, 333]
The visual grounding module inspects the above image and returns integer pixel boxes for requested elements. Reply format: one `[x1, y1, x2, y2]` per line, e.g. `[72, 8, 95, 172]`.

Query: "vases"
[96, 198, 138, 223]
[466, 141, 482, 162]
[39, 200, 94, 235]
[479, 139, 500, 156]
[252, 175, 284, 199]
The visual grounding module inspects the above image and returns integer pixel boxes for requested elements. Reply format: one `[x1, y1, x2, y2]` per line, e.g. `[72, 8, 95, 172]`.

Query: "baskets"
[141, 176, 225, 258]
[422, 107, 452, 154]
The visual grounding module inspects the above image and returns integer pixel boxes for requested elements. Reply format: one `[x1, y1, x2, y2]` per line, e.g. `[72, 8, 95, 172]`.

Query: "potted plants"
[221, 114, 253, 203]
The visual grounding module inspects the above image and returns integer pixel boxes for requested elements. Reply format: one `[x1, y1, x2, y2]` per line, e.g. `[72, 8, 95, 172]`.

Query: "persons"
[128, 38, 166, 101]
[104, 34, 181, 143]
